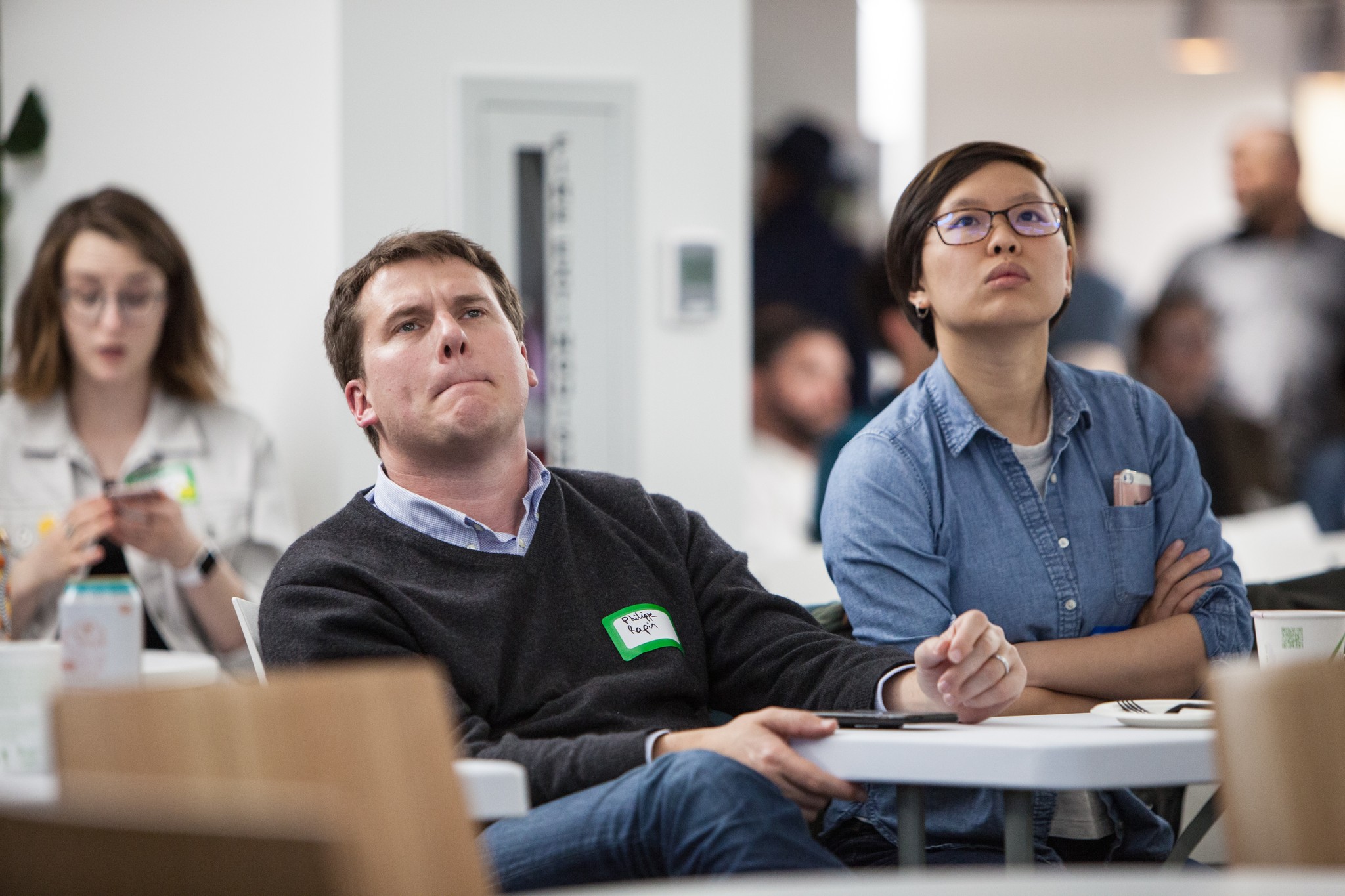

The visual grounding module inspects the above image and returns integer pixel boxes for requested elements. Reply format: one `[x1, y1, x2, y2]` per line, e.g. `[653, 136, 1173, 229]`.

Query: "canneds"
[57, 575, 142, 691]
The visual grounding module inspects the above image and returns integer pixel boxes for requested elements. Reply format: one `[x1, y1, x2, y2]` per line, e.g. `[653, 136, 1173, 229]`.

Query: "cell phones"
[108, 484, 158, 516]
[812, 707, 957, 723]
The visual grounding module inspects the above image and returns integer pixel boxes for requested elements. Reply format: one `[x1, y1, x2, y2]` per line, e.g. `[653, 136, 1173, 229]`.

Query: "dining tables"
[748, 717, 1216, 873]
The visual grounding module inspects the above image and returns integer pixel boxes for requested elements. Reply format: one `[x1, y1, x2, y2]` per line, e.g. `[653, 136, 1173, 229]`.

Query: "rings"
[992, 653, 1010, 676]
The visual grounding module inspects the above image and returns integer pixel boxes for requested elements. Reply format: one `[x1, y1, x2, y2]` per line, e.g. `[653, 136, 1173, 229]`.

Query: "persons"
[755, 121, 1345, 612]
[818, 143, 1252, 870]
[0, 188, 296, 682]
[257, 231, 1027, 896]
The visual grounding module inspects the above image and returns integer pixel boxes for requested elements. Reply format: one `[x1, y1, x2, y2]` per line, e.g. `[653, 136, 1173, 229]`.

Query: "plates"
[1090, 699, 1216, 728]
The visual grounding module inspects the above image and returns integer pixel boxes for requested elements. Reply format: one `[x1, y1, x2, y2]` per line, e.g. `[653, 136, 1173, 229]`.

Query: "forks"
[1117, 699, 1215, 713]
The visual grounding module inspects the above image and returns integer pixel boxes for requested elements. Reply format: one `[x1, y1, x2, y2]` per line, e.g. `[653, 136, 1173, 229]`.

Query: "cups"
[1250, 610, 1345, 670]
[0, 642, 66, 777]
[1205, 653, 1345, 876]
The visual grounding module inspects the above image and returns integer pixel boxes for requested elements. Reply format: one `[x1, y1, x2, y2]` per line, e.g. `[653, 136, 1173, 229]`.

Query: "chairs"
[1202, 661, 1345, 870]
[0, 644, 488, 896]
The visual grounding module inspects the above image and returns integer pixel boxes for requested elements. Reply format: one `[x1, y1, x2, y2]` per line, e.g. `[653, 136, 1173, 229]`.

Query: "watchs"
[174, 546, 217, 587]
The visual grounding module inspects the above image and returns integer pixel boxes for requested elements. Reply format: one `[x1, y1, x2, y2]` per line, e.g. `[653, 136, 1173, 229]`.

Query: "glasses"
[68, 286, 161, 314]
[920, 200, 1069, 246]
